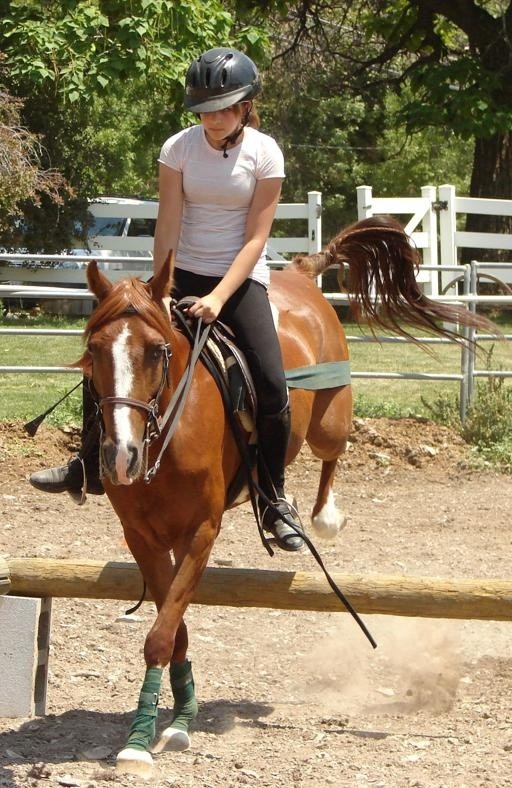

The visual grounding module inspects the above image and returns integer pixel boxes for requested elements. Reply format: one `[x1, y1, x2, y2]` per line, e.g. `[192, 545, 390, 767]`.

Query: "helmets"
[182, 46, 263, 114]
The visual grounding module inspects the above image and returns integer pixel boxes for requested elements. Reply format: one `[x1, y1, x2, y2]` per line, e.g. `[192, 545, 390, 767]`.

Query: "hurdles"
[3, 555, 511, 721]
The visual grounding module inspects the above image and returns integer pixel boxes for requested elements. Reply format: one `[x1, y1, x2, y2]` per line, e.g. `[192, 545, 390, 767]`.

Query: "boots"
[28, 374, 107, 496]
[255, 385, 305, 552]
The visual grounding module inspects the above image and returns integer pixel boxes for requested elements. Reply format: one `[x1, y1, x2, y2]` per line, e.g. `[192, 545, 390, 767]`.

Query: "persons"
[27, 44, 308, 551]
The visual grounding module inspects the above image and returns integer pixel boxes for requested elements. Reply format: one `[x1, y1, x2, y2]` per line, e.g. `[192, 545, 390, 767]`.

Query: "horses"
[63, 214, 508, 763]
